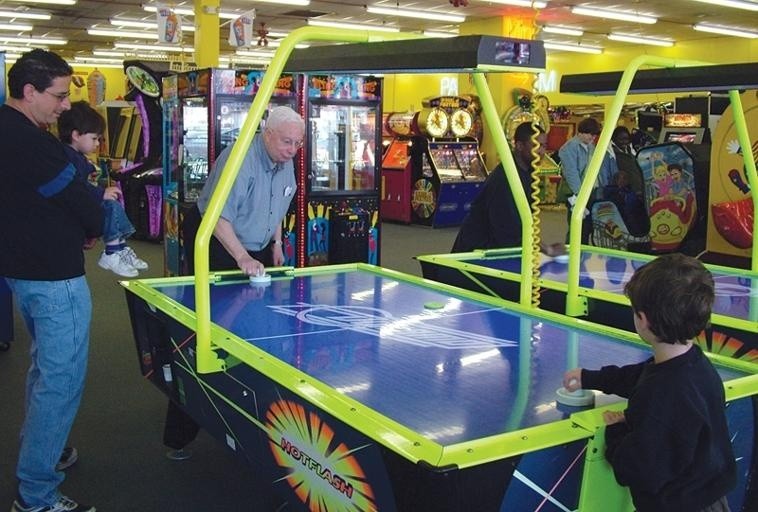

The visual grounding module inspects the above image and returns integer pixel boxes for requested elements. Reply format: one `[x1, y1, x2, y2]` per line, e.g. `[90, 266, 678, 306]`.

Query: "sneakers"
[55, 447, 78, 470]
[98, 250, 139, 278]
[122, 246, 149, 270]
[12, 492, 96, 512]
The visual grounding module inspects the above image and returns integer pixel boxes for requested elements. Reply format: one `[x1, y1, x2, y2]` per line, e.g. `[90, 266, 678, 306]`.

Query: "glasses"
[278, 135, 304, 150]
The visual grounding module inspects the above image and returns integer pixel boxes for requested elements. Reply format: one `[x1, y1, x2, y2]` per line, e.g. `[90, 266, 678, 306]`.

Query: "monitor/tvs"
[431, 148, 463, 183]
[381, 140, 411, 169]
[657, 127, 707, 148]
[455, 148, 487, 180]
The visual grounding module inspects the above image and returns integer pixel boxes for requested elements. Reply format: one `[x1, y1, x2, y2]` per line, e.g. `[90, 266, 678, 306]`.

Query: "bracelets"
[271, 239, 283, 245]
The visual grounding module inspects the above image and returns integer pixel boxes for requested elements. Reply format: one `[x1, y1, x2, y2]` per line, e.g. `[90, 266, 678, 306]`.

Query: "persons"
[1, 46, 95, 512]
[558, 116, 649, 244]
[449, 122, 567, 256]
[563, 251, 732, 512]
[182, 103, 305, 276]
[59, 98, 148, 278]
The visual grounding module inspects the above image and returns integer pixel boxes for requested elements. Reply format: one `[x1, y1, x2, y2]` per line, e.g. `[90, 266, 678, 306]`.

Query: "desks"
[112, 255, 756, 511]
[412, 239, 757, 369]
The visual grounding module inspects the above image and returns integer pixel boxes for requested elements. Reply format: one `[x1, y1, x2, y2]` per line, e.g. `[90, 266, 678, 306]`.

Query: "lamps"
[0, 1, 758, 98]
